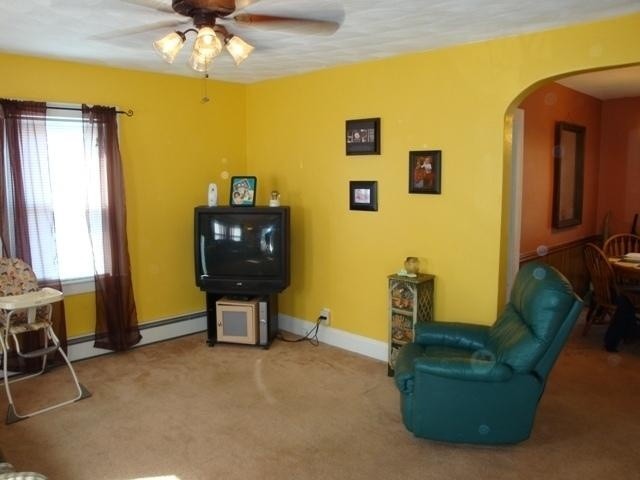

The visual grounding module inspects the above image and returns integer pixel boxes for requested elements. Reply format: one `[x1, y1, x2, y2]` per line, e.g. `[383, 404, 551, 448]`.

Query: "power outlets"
[321, 310, 330, 327]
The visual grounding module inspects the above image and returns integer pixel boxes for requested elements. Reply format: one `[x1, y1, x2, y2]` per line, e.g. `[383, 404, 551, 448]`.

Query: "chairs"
[583, 242, 622, 353]
[0, 257, 92, 426]
[603, 233, 640, 257]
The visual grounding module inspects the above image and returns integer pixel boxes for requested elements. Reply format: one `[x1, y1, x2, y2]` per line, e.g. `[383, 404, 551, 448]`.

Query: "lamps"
[153, 14, 254, 106]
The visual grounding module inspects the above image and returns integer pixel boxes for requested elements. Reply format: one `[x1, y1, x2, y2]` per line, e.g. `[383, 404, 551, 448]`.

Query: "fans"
[90, 0, 339, 43]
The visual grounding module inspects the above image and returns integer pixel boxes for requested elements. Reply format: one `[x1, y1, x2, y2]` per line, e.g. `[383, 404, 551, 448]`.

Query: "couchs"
[395, 260, 584, 447]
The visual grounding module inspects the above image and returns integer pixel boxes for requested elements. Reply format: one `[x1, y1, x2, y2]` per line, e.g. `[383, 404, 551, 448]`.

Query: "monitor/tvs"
[193, 205, 292, 290]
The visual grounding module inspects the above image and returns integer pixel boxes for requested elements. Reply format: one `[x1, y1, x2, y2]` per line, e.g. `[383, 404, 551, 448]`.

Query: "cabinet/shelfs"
[203, 289, 276, 347]
[387, 273, 435, 379]
[215, 296, 262, 345]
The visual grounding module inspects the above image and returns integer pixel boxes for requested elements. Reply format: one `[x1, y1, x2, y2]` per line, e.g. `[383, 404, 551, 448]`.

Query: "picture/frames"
[345, 118, 381, 156]
[230, 176, 257, 207]
[409, 150, 442, 195]
[551, 120, 587, 230]
[349, 180, 378, 211]
